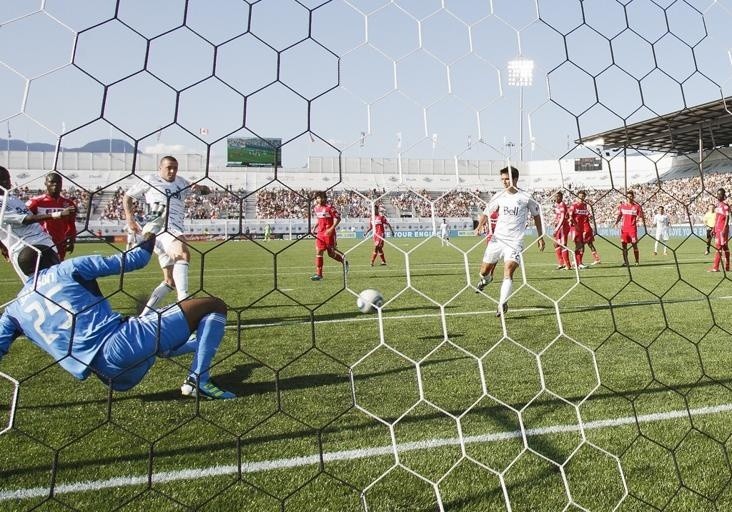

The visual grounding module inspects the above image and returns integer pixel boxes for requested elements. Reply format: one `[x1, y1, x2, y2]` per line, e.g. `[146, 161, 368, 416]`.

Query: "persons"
[472, 163, 547, 319]
[184, 183, 318, 218]
[325, 188, 496, 218]
[649, 203, 673, 258]
[478, 202, 500, 246]
[567, 191, 588, 270]
[578, 213, 600, 268]
[22, 172, 78, 263]
[262, 223, 274, 243]
[437, 217, 451, 245]
[227, 136, 283, 164]
[0, 216, 241, 407]
[702, 202, 717, 255]
[362, 203, 395, 268]
[122, 155, 211, 318]
[704, 187, 731, 273]
[0, 165, 78, 287]
[525, 171, 730, 225]
[550, 191, 572, 273]
[9, 184, 92, 216]
[609, 188, 647, 269]
[307, 190, 350, 283]
[101, 186, 144, 216]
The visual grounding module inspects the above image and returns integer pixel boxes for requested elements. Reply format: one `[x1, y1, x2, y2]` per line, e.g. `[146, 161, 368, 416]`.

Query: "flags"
[198, 127, 209, 136]
[310, 134, 316, 143]
[357, 131, 474, 150]
[6, 121, 12, 138]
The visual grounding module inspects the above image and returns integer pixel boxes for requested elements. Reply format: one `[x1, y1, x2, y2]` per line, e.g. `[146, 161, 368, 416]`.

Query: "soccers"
[357, 288, 384, 314]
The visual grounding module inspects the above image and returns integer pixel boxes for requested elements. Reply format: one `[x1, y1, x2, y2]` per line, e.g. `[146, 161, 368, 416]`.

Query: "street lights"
[506, 59, 536, 160]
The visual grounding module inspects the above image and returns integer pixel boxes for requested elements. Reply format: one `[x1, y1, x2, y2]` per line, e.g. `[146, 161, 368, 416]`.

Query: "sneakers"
[380, 262, 387, 265]
[496, 302, 508, 318]
[653, 252, 658, 256]
[591, 260, 601, 265]
[704, 250, 710, 255]
[343, 265, 352, 275]
[622, 262, 628, 267]
[370, 264, 374, 267]
[180, 377, 238, 400]
[663, 253, 668, 256]
[633, 262, 640, 267]
[475, 276, 493, 294]
[725, 260, 730, 271]
[704, 266, 720, 272]
[310, 275, 323, 281]
[556, 264, 589, 271]
